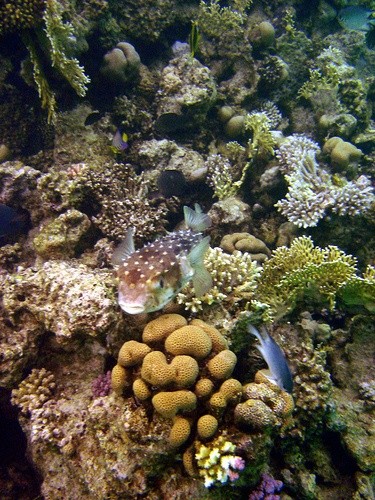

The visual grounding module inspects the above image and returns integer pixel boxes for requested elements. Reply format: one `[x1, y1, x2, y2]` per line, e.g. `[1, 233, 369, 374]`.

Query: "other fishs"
[109, 122, 135, 153]
[108, 203, 210, 314]
[245, 322, 293, 393]
[187, 18, 200, 64]
